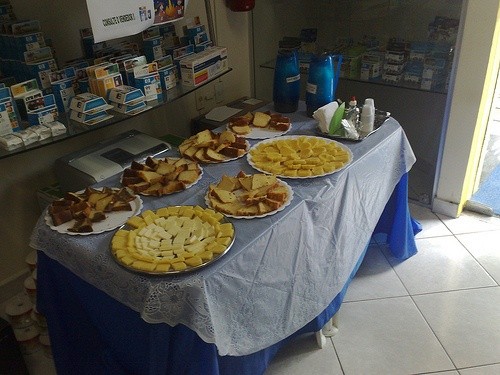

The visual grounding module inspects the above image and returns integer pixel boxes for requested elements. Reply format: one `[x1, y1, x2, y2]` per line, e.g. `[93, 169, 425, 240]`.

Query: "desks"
[28, 97, 402, 375]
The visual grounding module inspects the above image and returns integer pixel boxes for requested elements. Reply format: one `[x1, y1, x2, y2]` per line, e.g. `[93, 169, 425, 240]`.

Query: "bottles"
[272, 36, 302, 113]
[306, 48, 343, 119]
[345, 97, 360, 131]
[360, 104, 374, 133]
[364, 98, 376, 119]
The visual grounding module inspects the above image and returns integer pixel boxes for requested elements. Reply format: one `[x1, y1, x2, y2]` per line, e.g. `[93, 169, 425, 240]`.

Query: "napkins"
[313, 101, 339, 133]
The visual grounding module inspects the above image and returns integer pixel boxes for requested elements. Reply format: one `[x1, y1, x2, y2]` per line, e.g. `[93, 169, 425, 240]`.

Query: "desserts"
[250, 136, 349, 177]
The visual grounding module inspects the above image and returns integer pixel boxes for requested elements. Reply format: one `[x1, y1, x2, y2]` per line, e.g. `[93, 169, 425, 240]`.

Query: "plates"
[110, 204, 237, 275]
[247, 135, 354, 179]
[177, 132, 252, 164]
[225, 114, 292, 140]
[204, 174, 294, 220]
[119, 157, 204, 196]
[44, 187, 144, 236]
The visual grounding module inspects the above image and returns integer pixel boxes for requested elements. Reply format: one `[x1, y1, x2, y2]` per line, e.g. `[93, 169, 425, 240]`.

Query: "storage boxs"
[340, 33, 457, 91]
[0, 0, 229, 152]
[56, 129, 169, 193]
[190, 96, 262, 137]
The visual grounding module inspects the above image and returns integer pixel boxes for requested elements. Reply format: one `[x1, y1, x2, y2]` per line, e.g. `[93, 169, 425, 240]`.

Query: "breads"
[178, 129, 247, 162]
[122, 156, 202, 196]
[207, 171, 288, 216]
[48, 186, 136, 232]
[228, 109, 291, 134]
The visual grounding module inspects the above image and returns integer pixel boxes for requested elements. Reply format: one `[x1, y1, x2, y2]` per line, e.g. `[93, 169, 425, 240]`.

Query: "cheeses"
[111, 205, 232, 273]
